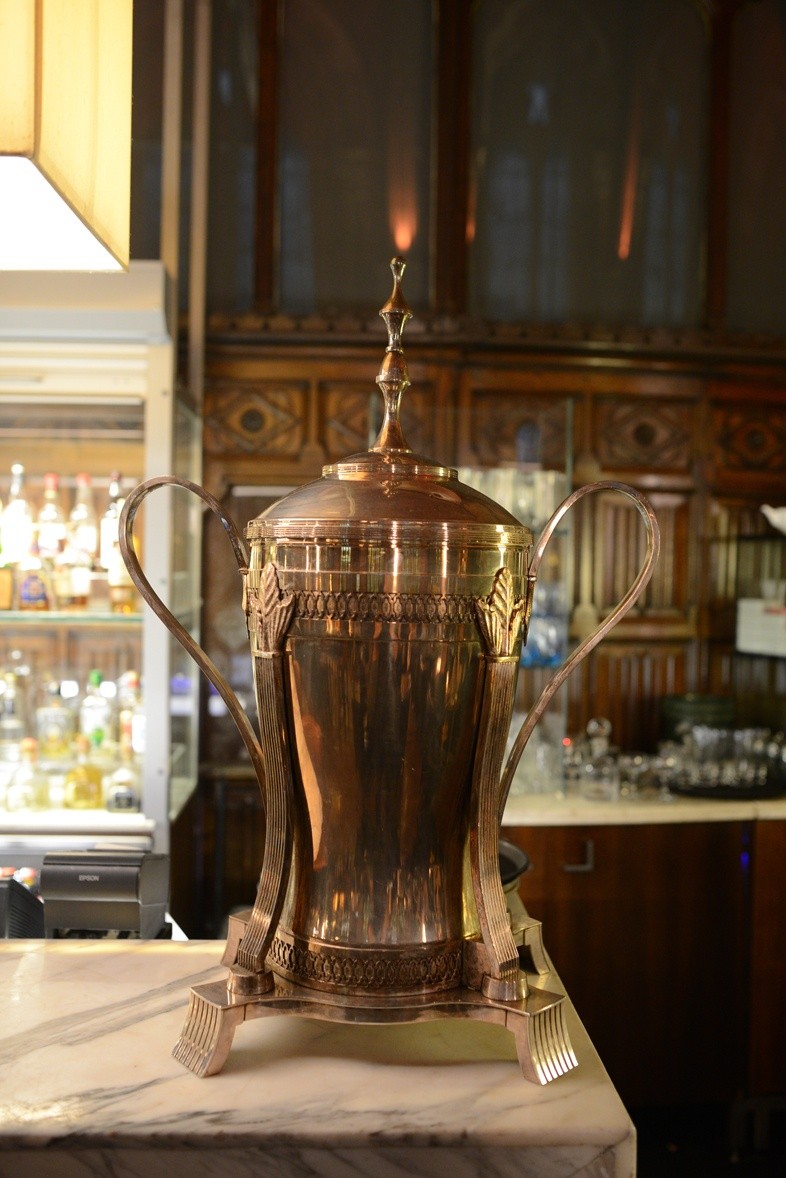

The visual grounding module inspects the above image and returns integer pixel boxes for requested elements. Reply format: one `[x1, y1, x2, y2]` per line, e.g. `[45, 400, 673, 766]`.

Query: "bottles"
[0, 466, 141, 614]
[1, 649, 141, 813]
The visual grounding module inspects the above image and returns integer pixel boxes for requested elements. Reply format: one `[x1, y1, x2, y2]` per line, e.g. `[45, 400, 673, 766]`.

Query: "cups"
[579, 759, 615, 801]
[659, 726, 786, 785]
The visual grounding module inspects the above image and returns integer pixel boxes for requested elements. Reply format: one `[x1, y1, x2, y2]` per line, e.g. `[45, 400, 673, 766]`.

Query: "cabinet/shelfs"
[0, 337, 201, 824]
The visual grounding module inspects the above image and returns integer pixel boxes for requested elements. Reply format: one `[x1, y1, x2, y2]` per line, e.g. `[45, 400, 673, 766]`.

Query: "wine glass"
[649, 756, 684, 801]
[618, 751, 651, 801]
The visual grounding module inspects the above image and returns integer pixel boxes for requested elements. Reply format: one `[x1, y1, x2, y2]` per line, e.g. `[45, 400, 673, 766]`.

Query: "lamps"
[1, 1, 133, 272]
